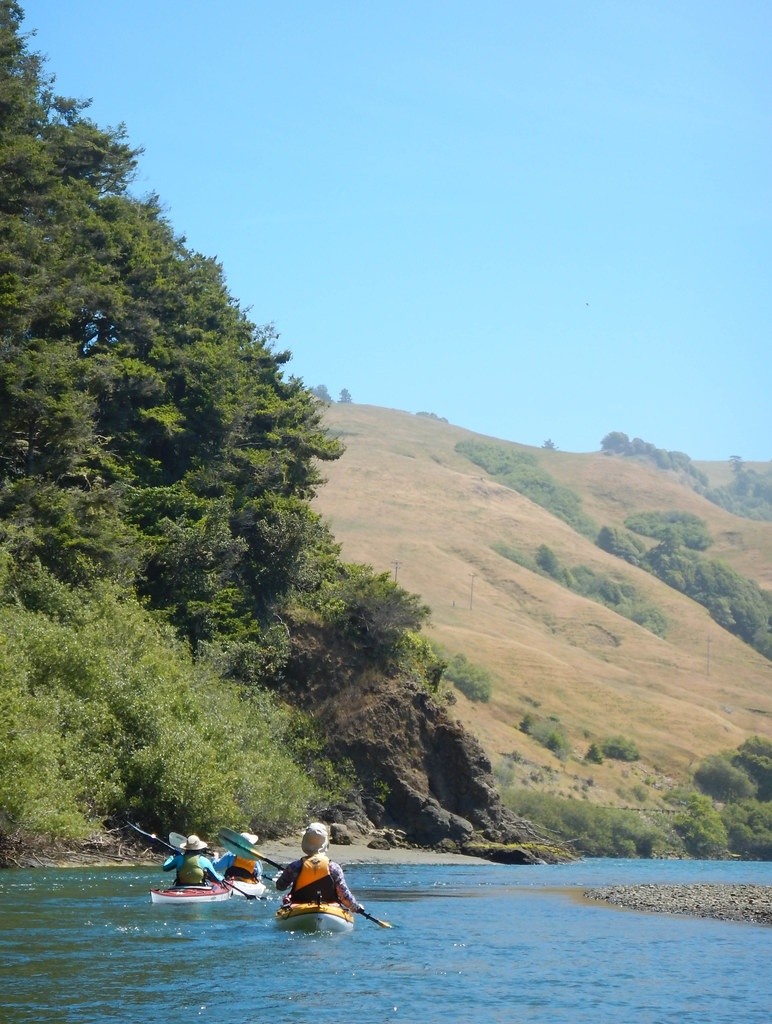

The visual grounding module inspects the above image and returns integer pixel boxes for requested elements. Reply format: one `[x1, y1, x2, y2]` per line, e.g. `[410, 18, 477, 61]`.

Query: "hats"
[180, 835, 207, 850]
[301, 823, 330, 856]
[240, 832, 259, 844]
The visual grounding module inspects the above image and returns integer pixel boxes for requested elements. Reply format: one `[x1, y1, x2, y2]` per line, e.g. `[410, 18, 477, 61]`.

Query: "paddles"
[169, 832, 272, 880]
[126, 820, 267, 901]
[217, 825, 393, 929]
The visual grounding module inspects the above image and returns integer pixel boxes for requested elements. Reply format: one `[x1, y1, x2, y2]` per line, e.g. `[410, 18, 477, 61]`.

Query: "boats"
[228, 880, 266, 898]
[149, 876, 235, 905]
[273, 891, 356, 933]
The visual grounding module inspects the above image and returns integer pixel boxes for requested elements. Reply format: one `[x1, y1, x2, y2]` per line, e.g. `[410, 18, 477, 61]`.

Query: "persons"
[162, 823, 365, 914]
